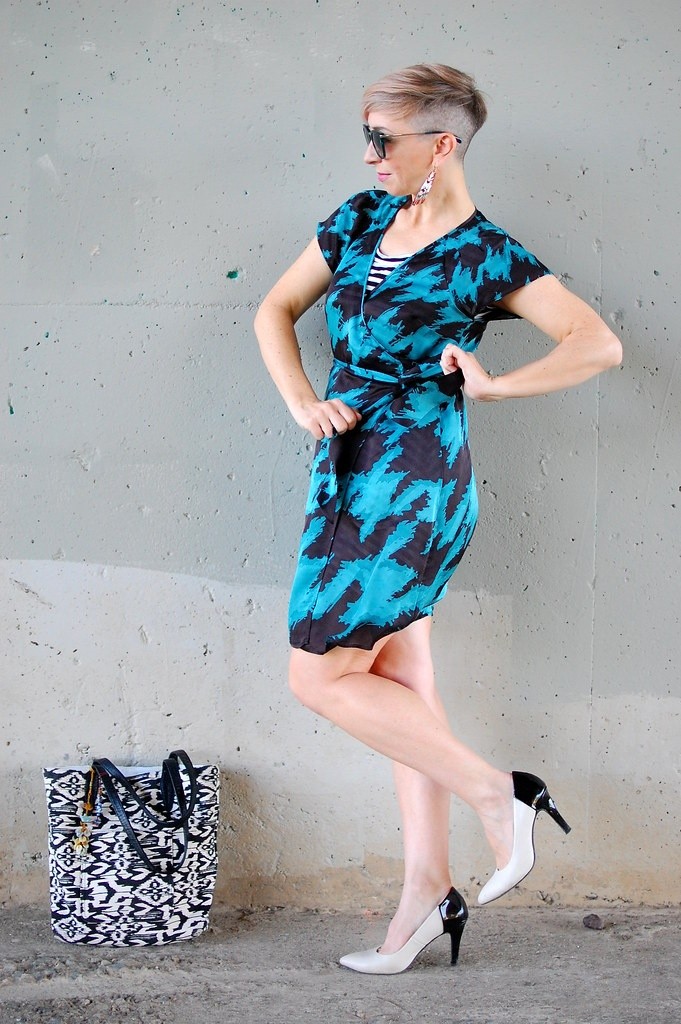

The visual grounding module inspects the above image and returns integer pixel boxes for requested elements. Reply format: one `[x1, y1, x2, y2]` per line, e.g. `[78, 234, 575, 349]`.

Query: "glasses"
[361, 122, 462, 159]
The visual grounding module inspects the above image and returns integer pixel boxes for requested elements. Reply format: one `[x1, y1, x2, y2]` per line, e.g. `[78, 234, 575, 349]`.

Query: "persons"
[252, 63, 624, 976]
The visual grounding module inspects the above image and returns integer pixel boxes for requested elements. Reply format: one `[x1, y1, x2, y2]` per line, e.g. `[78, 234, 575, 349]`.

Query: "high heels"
[477, 771, 571, 905]
[340, 886, 469, 974]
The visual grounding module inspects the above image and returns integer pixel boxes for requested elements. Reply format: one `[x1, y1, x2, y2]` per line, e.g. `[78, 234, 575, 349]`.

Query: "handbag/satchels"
[43, 750, 219, 948]
